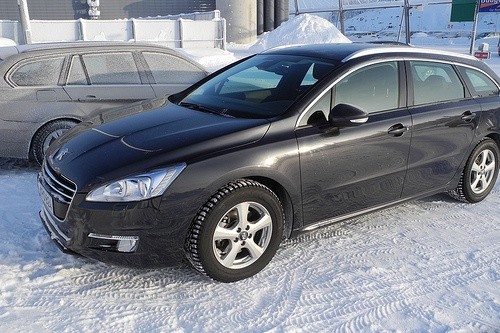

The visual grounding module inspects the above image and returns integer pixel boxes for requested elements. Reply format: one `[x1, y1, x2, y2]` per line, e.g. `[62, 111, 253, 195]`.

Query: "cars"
[0, 39, 264, 174]
[36, 42, 500, 285]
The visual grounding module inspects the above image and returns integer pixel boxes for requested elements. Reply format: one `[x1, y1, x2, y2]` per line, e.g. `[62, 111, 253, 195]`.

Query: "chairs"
[424, 75, 448, 98]
[312, 60, 335, 79]
